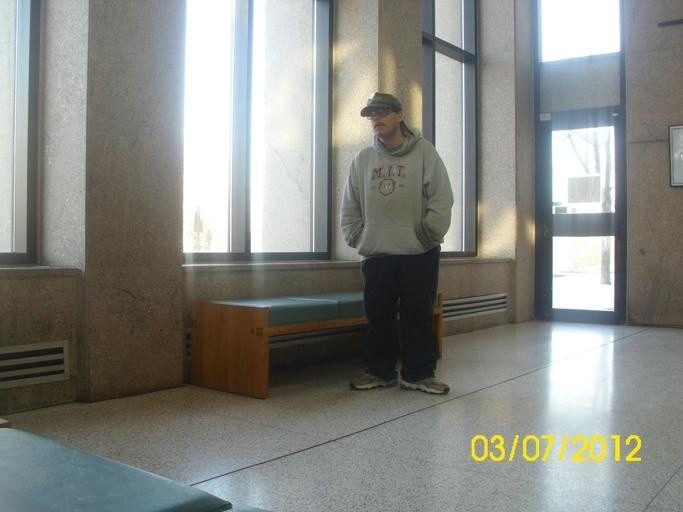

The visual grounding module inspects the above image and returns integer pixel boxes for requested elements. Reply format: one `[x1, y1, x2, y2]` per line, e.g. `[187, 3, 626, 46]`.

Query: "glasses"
[366, 111, 393, 117]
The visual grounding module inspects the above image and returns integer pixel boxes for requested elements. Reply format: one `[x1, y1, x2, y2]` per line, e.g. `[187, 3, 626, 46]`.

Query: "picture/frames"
[669, 125, 683, 188]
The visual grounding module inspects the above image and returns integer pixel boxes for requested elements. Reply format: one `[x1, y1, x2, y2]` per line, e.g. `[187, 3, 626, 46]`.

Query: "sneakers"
[400, 377, 450, 394]
[348, 371, 398, 389]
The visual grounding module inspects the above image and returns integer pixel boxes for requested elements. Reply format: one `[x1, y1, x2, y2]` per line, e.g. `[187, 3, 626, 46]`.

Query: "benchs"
[188, 290, 442, 400]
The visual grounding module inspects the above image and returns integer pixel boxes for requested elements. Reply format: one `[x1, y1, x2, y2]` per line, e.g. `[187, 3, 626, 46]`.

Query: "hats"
[360, 92, 402, 117]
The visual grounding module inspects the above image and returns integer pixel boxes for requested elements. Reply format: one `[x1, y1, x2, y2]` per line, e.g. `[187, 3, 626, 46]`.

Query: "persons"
[339, 90, 454, 396]
